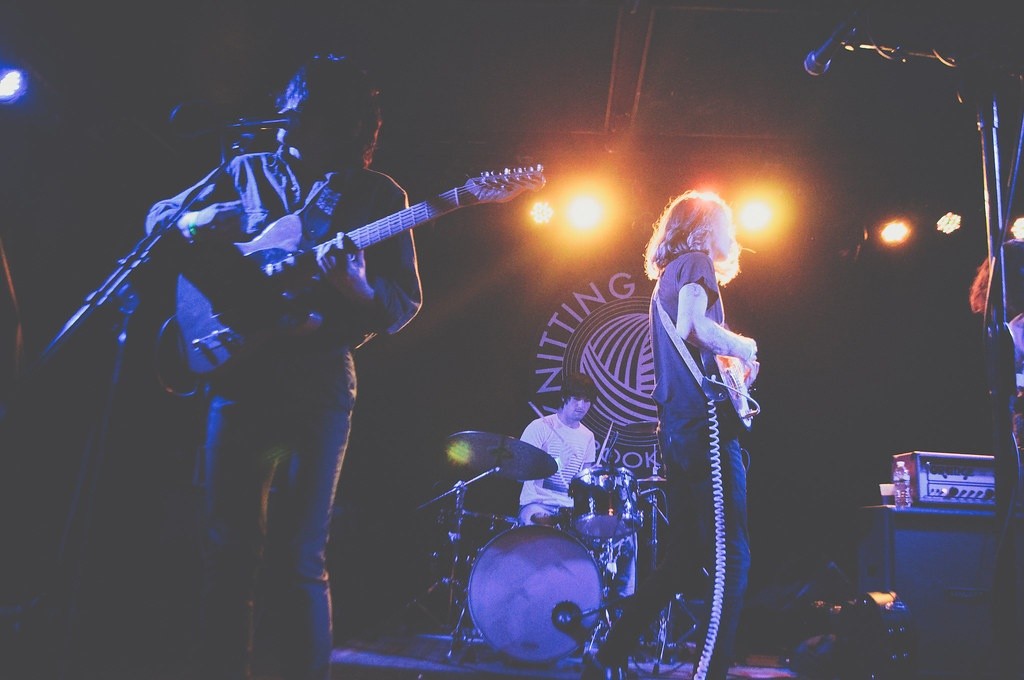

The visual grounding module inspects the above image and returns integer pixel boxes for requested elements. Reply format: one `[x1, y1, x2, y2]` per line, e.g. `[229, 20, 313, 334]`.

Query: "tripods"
[388, 468, 503, 637]
[651, 495, 697, 677]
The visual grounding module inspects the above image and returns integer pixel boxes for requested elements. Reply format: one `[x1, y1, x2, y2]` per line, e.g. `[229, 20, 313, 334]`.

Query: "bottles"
[892, 461, 912, 507]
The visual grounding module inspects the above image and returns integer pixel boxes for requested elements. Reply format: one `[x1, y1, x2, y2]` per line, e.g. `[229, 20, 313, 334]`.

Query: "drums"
[566, 467, 644, 538]
[465, 523, 613, 664]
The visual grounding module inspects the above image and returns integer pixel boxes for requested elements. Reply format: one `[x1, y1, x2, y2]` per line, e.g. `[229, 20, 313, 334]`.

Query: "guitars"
[703, 335, 760, 439]
[144, 162, 547, 397]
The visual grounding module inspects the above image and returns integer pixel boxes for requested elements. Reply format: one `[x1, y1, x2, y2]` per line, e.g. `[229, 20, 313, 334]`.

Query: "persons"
[144, 56, 423, 680]
[517, 372, 597, 535]
[642, 186, 759, 680]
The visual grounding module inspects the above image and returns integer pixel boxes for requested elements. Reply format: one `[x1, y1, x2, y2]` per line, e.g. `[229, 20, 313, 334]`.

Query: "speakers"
[859, 505, 1001, 680]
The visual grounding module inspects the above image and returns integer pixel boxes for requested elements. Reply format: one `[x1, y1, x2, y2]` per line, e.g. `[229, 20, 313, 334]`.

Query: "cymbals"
[639, 475, 669, 484]
[444, 427, 559, 484]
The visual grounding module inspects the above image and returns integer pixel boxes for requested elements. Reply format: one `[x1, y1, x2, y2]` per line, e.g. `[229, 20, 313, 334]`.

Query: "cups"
[879, 483, 894, 505]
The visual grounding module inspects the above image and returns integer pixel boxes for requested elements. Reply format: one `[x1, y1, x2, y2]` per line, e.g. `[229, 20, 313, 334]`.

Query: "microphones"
[555, 610, 574, 627]
[223, 106, 301, 132]
[805, 20, 855, 76]
[639, 487, 660, 497]
